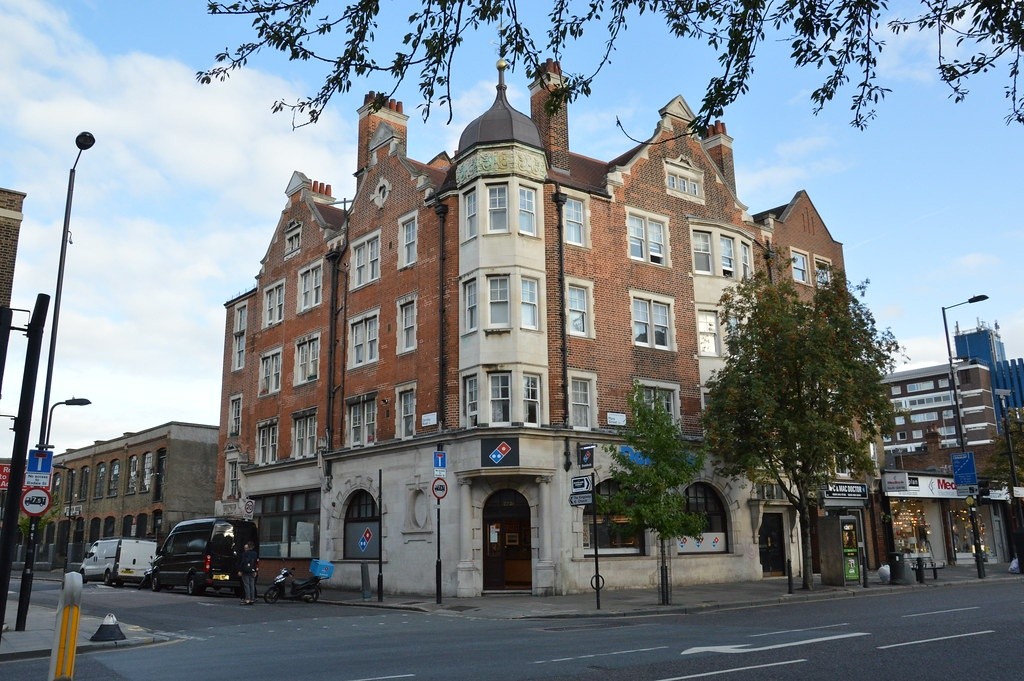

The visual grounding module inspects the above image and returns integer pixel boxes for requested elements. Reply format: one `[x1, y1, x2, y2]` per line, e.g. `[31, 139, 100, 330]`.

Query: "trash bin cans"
[889, 552, 907, 585]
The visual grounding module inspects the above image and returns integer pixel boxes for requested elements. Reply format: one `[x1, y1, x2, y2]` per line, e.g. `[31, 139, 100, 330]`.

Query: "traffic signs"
[571, 476, 592, 493]
[568, 491, 593, 506]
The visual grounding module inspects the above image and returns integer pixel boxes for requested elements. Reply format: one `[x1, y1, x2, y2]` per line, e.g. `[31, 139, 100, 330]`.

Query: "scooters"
[264, 559, 329, 604]
[137, 556, 154, 590]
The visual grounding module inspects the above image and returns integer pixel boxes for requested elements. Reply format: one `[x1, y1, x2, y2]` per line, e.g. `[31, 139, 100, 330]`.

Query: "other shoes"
[240, 601, 254, 605]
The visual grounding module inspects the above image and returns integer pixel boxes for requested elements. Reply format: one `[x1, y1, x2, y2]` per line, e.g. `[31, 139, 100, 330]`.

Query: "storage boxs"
[310, 559, 334, 578]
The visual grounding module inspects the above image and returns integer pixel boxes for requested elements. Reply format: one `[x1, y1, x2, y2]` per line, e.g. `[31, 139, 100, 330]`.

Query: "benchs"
[911, 560, 945, 581]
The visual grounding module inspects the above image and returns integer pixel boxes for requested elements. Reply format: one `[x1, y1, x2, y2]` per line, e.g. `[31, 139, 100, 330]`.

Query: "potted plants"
[882, 515, 891, 524]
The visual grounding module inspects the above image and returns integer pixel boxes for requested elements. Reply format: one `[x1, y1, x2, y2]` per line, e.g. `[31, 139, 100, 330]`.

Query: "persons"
[238, 541, 259, 606]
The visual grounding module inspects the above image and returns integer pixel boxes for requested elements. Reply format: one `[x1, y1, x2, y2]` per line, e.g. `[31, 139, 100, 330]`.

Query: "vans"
[151, 516, 259, 599]
[79, 536, 158, 587]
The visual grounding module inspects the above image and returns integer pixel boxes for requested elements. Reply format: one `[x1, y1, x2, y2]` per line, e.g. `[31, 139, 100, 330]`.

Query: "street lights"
[10, 132, 95, 631]
[942, 295, 990, 579]
